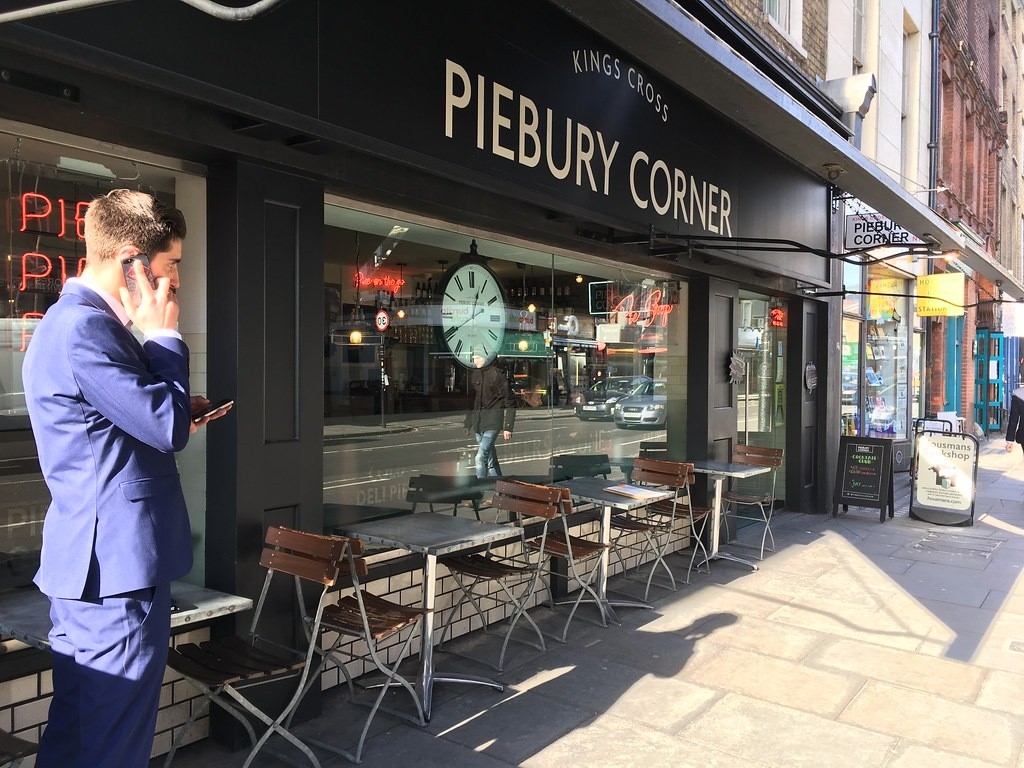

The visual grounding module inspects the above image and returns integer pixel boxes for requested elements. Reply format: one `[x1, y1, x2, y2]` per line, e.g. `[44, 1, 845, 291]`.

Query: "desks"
[678, 459, 771, 571]
[547, 477, 677, 625]
[0, 580, 254, 650]
[334, 512, 523, 723]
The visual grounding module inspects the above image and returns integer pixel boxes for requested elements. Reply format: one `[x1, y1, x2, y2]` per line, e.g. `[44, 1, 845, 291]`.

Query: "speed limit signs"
[376, 310, 389, 331]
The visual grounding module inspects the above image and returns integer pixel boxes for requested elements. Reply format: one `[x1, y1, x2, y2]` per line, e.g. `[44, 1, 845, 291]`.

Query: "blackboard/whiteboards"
[832, 435, 895, 509]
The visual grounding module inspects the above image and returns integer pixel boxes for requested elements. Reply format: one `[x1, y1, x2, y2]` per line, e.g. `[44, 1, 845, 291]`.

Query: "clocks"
[431, 238, 511, 373]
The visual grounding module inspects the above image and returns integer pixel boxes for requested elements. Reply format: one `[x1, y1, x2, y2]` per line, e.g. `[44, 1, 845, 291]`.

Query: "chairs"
[165, 526, 434, 768]
[719, 444, 785, 560]
[608, 456, 716, 609]
[437, 480, 607, 671]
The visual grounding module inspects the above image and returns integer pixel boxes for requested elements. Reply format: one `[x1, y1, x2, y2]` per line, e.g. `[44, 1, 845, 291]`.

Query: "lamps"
[516, 262, 536, 351]
[348, 231, 364, 344]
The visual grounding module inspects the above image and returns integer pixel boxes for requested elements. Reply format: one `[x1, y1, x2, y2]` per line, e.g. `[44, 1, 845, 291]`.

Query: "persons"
[463, 345, 516, 507]
[22, 188, 233, 768]
[1006, 386, 1024, 452]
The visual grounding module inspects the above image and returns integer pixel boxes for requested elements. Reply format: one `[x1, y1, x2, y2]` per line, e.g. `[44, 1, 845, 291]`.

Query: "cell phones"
[192, 399, 234, 422]
[120, 254, 156, 308]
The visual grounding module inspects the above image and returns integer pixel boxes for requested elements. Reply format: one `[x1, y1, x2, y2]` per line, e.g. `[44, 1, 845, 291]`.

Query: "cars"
[573, 375, 652, 421]
[614, 378, 667, 428]
